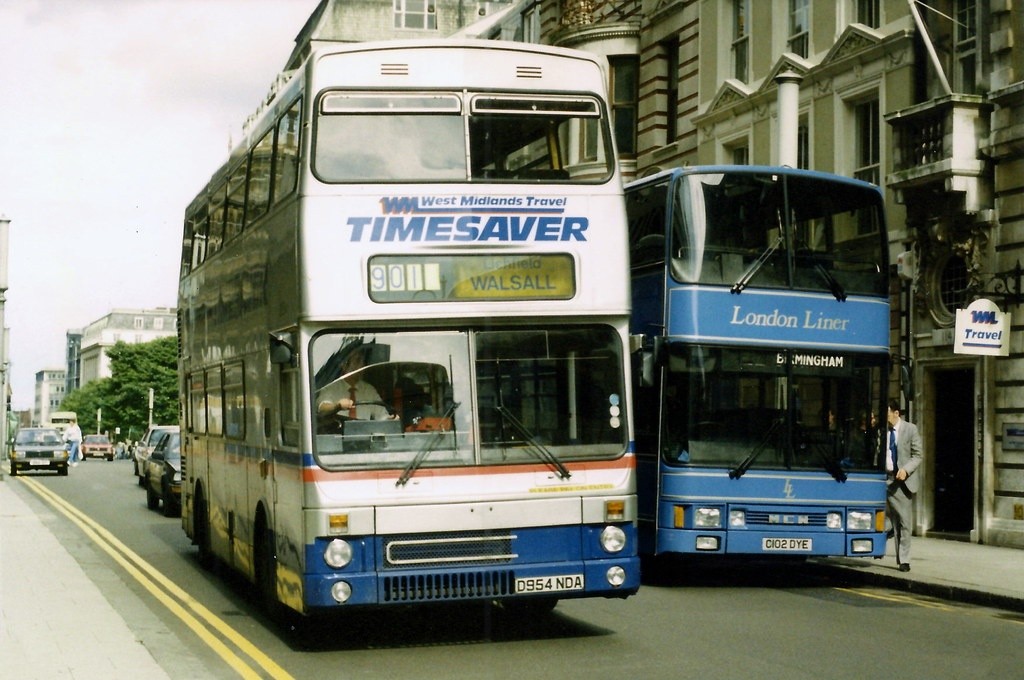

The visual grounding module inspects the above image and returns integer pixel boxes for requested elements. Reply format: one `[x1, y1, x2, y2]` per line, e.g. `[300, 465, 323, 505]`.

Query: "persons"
[316, 347, 401, 426]
[872, 397, 924, 572]
[814, 399, 875, 468]
[116, 436, 132, 459]
[63, 419, 82, 468]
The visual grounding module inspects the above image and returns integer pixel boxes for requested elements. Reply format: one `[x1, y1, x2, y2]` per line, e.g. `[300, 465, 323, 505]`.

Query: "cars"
[81, 434, 114, 462]
[5, 428, 72, 477]
[131, 425, 179, 489]
[143, 431, 183, 518]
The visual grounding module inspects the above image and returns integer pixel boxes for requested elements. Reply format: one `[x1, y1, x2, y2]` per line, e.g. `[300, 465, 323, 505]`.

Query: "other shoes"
[900, 564, 910, 572]
[67, 461, 78, 467]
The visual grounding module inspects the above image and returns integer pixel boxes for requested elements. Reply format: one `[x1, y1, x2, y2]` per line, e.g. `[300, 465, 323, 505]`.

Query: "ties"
[348, 388, 356, 419]
[889, 427, 897, 464]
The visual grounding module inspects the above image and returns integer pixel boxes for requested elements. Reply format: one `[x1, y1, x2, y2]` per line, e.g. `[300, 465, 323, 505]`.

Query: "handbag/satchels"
[77, 445, 84, 460]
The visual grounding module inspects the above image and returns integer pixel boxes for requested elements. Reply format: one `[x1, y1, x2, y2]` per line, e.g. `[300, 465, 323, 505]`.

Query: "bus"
[176, 37, 642, 631]
[622, 165, 915, 584]
[49, 412, 77, 438]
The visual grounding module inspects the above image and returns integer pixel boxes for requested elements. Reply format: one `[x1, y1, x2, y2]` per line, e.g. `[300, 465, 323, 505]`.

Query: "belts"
[887, 470, 895, 476]
